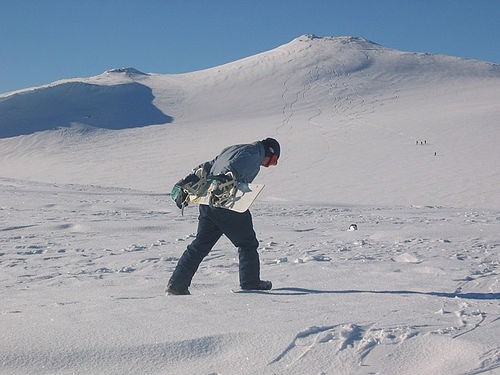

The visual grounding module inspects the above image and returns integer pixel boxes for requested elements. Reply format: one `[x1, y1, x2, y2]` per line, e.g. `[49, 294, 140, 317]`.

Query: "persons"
[166, 137, 280, 295]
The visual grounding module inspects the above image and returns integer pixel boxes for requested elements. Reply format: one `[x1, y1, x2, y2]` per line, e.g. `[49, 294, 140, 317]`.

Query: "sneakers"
[166, 279, 191, 295]
[239, 280, 272, 290]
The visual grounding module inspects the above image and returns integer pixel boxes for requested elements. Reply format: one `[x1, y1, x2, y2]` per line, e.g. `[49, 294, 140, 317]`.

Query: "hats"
[263, 138, 280, 159]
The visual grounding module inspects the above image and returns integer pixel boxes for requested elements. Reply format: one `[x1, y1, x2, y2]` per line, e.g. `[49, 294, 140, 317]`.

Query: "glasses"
[266, 147, 277, 167]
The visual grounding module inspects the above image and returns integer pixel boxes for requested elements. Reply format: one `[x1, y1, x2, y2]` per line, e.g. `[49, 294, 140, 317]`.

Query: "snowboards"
[186, 176, 265, 213]
